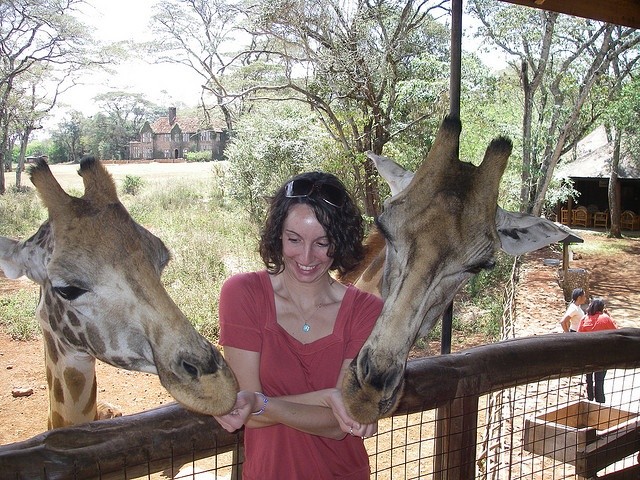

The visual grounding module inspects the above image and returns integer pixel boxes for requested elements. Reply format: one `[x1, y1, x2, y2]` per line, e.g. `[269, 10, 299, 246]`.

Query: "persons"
[560, 287, 587, 333]
[211, 170, 384, 480]
[577, 298, 617, 403]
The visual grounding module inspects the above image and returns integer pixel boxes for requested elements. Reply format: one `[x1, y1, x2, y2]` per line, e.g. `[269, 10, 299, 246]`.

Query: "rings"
[350, 428, 355, 437]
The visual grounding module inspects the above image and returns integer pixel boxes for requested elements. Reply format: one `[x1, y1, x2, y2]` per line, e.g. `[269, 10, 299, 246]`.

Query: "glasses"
[283, 179, 348, 208]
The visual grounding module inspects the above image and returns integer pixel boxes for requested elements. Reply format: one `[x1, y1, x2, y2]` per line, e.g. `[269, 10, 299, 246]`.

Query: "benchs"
[561, 206, 592, 228]
[621, 211, 638, 230]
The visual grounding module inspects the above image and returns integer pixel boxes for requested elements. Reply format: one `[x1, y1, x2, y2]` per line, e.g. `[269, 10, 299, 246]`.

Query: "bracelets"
[250, 390, 268, 417]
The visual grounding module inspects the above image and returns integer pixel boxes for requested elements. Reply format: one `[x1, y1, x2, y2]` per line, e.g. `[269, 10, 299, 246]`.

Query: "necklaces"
[282, 272, 331, 333]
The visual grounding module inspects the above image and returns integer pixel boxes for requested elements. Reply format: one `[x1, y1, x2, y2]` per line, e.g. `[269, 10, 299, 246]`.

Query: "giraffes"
[1, 153, 238, 433]
[334, 113, 585, 425]
